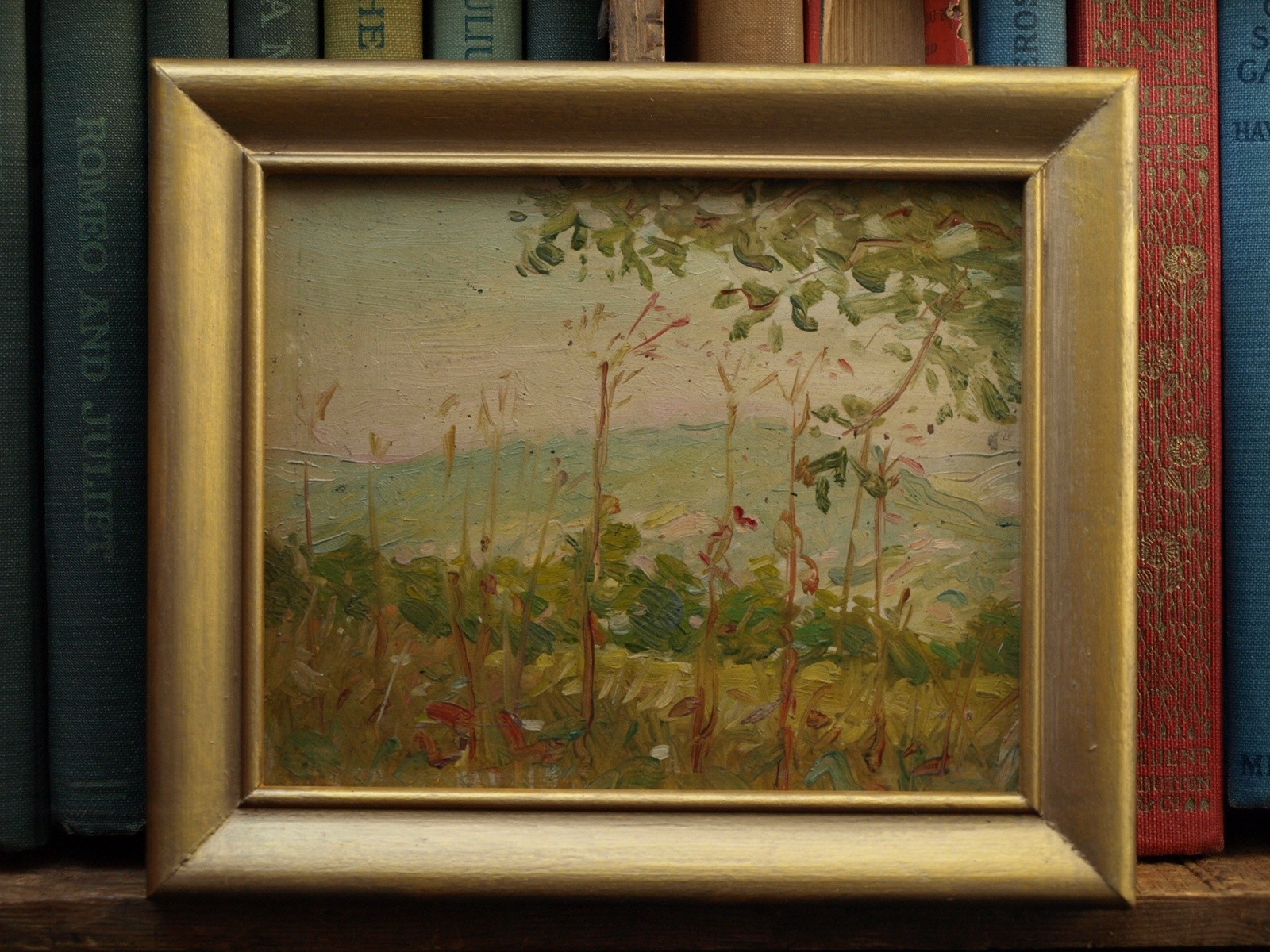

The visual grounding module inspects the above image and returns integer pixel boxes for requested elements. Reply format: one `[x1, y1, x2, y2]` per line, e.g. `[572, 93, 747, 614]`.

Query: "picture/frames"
[150, 61, 1140, 902]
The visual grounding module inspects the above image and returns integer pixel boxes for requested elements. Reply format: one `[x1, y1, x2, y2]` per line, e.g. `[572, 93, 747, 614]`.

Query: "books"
[0, 1, 1270, 868]
